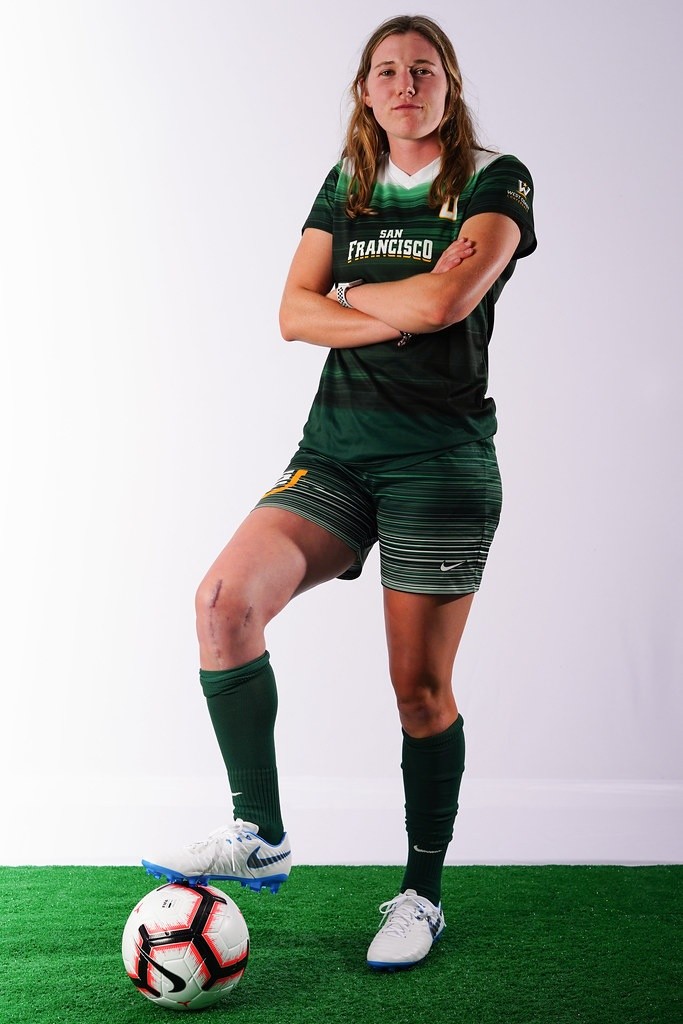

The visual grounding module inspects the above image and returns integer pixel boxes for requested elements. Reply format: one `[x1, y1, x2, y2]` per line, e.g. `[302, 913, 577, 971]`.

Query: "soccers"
[120, 881, 250, 1012]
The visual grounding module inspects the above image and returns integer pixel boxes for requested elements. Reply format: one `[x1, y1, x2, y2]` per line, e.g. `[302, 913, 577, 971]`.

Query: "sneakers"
[139, 818, 293, 895]
[363, 886, 447, 974]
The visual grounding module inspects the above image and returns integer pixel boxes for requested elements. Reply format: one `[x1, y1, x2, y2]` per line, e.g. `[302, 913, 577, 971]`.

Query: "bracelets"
[394, 331, 416, 347]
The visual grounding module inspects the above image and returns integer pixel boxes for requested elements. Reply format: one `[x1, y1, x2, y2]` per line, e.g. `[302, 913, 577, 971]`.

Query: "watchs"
[337, 279, 364, 309]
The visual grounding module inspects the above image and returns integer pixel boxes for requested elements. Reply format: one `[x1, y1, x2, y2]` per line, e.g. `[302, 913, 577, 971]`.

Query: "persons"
[140, 14, 536, 973]
